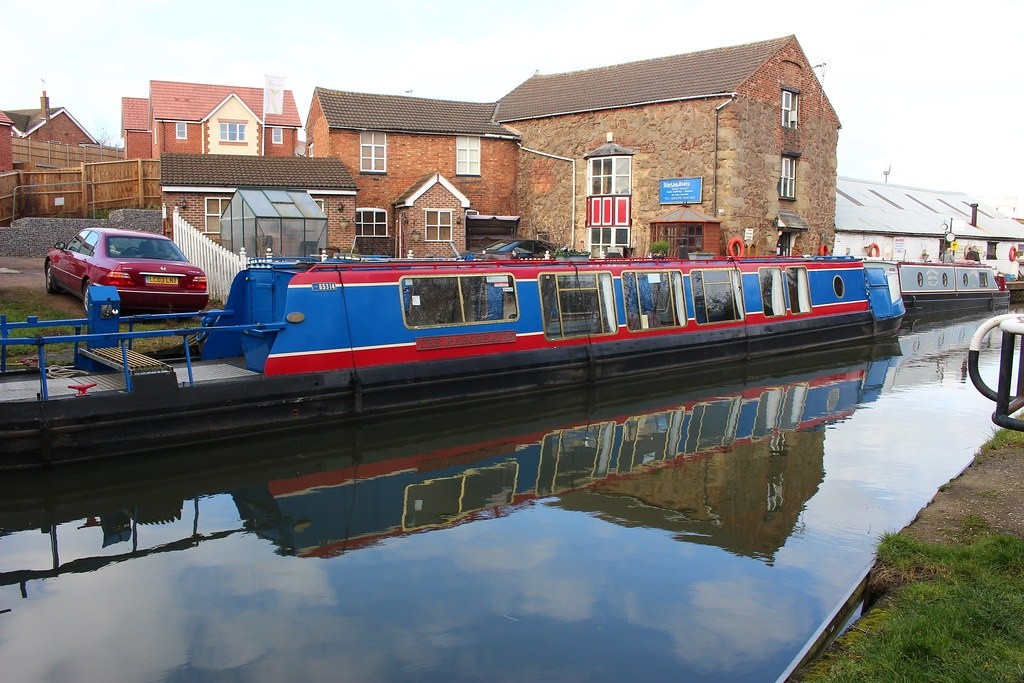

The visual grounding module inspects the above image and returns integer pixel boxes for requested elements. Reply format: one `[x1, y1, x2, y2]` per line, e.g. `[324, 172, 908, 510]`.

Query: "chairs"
[140, 241, 155, 253]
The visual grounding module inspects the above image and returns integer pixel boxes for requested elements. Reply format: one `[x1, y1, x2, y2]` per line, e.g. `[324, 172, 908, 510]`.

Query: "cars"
[44, 227, 210, 320]
[453, 240, 554, 258]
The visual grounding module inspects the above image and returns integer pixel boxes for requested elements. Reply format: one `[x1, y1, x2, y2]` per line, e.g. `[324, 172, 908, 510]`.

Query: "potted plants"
[687, 246, 715, 262]
[651, 240, 670, 258]
[554, 245, 592, 265]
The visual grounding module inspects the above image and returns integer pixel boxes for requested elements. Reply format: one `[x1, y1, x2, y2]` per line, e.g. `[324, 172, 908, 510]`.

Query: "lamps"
[337, 202, 344, 213]
[864, 245, 871, 255]
[181, 197, 186, 209]
[922, 250, 930, 261]
[1015, 251, 1023, 258]
[457, 215, 461, 224]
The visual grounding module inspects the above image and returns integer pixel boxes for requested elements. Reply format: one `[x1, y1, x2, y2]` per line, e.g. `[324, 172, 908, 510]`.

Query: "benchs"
[80, 346, 178, 392]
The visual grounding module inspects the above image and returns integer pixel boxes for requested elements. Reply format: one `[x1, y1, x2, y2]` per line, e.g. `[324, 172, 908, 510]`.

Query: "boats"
[897, 262, 1011, 316]
[0, 257, 907, 471]
[0, 336, 905, 601]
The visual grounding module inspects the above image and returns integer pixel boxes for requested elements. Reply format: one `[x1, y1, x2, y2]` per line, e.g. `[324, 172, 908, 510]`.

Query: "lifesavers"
[747, 243, 759, 257]
[1009, 245, 1016, 260]
[819, 243, 827, 256]
[869, 243, 880, 257]
[728, 237, 745, 260]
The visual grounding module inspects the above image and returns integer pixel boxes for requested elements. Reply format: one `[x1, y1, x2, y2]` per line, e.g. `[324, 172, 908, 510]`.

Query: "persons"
[842, 247, 856, 259]
[966, 245, 981, 262]
[939, 247, 955, 264]
[921, 249, 930, 262]
